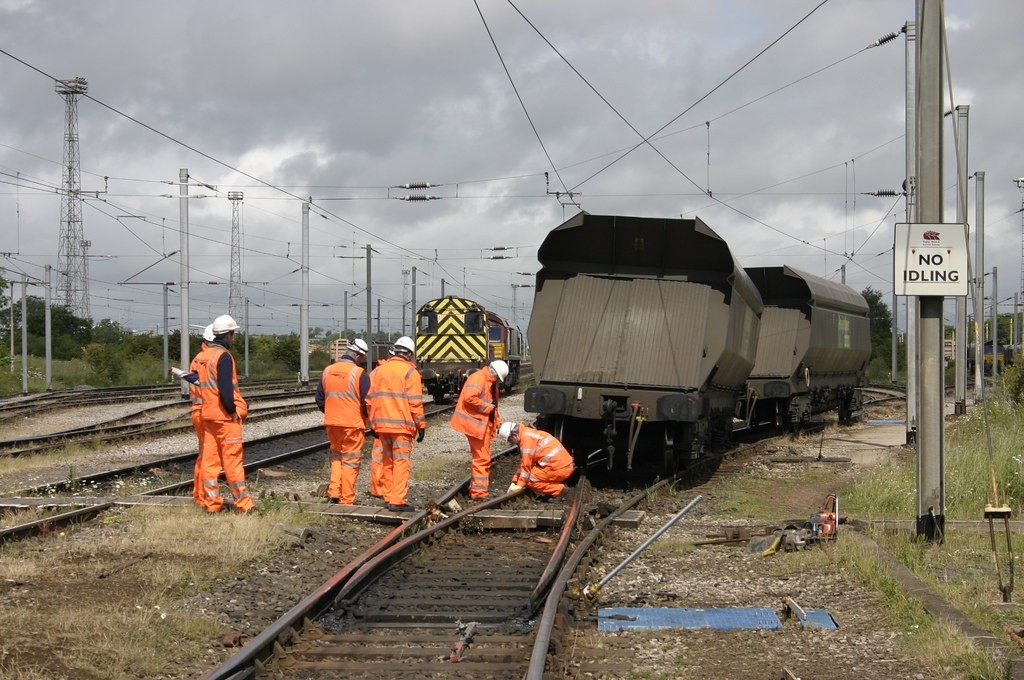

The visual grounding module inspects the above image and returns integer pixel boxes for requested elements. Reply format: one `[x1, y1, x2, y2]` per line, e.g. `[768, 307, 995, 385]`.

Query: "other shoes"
[389, 503, 415, 512]
[384, 501, 389, 508]
[327, 497, 339, 504]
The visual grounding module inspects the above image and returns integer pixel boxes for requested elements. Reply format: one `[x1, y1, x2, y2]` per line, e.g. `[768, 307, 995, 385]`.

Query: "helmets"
[394, 336, 415, 355]
[213, 315, 240, 335]
[347, 338, 368, 355]
[491, 359, 509, 382]
[203, 323, 217, 342]
[499, 422, 516, 443]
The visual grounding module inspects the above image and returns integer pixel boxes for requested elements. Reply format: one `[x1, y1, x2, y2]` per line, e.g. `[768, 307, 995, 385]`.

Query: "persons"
[198, 314, 263, 517]
[499, 421, 575, 503]
[364, 335, 427, 513]
[449, 359, 509, 500]
[314, 338, 371, 507]
[188, 323, 218, 509]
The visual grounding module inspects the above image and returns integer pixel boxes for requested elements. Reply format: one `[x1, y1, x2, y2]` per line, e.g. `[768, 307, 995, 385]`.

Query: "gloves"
[416, 428, 425, 443]
[507, 483, 515, 493]
[370, 428, 379, 438]
[364, 428, 371, 436]
[511, 485, 522, 491]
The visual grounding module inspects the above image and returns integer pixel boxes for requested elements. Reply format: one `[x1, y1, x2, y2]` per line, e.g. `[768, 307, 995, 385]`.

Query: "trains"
[524, 210, 872, 478]
[414, 295, 522, 407]
